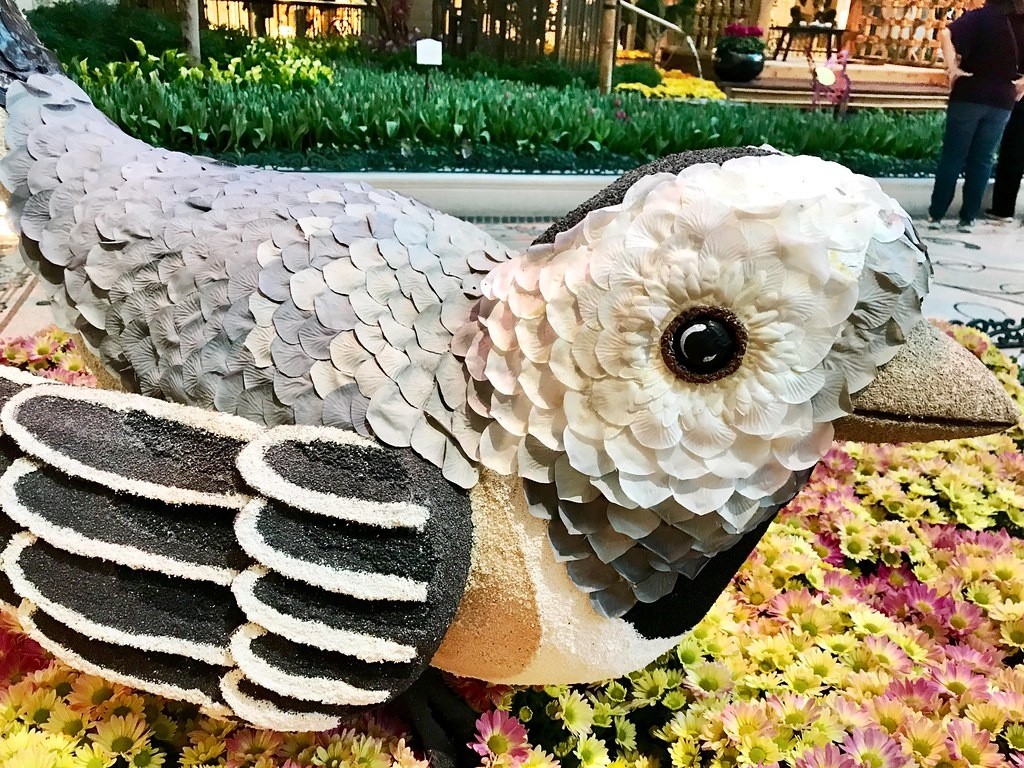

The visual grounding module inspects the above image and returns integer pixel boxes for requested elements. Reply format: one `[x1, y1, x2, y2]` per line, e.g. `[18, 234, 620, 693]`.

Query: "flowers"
[716, 21, 766, 50]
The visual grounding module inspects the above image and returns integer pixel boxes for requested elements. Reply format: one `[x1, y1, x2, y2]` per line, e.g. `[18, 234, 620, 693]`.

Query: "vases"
[712, 49, 765, 83]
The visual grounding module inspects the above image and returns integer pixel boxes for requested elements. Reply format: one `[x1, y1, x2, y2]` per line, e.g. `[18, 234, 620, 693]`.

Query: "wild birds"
[0, 2, 1018, 737]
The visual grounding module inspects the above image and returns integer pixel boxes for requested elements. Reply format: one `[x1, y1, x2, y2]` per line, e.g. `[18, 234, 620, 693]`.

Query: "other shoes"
[927, 218, 940, 229]
[956, 220, 975, 232]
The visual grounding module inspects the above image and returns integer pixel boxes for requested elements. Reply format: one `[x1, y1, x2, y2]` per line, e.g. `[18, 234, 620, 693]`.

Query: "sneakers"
[983, 208, 1014, 223]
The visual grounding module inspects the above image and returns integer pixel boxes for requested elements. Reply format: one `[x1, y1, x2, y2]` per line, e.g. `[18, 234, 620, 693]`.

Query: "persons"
[921, 0, 1024, 233]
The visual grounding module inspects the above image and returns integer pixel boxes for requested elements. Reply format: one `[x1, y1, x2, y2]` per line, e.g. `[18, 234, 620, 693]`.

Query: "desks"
[769, 25, 853, 61]
